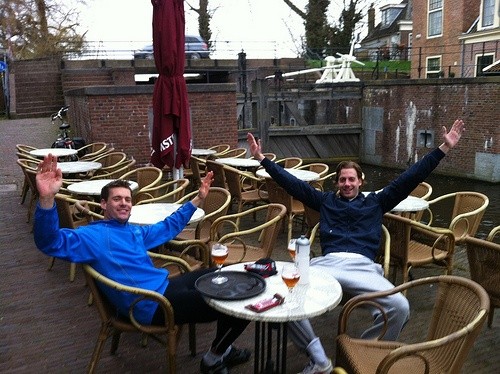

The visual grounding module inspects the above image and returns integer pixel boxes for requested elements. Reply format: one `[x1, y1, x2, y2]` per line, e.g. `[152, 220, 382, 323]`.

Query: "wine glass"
[282, 263, 301, 306]
[288, 239, 297, 263]
[211, 243, 229, 285]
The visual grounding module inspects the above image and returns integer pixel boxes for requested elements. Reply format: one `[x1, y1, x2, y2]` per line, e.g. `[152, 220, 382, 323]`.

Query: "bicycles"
[51, 104, 80, 161]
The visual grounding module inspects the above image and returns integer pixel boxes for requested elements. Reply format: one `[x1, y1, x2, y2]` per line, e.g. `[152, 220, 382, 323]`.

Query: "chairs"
[14, 143, 500, 374]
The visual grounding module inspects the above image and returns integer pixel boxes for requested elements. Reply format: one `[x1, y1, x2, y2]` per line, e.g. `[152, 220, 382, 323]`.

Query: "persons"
[247, 120, 466, 374]
[33, 153, 251, 374]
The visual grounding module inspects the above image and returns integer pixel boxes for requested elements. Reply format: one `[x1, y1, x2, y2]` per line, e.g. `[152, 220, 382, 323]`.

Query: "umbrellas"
[150, 0, 191, 191]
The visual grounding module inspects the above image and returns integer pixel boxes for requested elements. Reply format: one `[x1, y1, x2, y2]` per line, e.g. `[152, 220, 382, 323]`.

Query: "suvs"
[135, 35, 209, 60]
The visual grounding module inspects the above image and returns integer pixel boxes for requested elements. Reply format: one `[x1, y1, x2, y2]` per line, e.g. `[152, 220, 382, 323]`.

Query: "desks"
[203, 260, 344, 374]
[127, 203, 205, 228]
[256, 168, 320, 181]
[30, 148, 77, 156]
[192, 149, 214, 158]
[216, 157, 261, 170]
[68, 178, 139, 213]
[58, 162, 103, 178]
[362, 192, 430, 213]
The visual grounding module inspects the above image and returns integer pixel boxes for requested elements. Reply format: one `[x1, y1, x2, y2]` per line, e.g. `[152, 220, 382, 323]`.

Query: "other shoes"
[222, 346, 252, 367]
[296, 358, 333, 374]
[201, 355, 229, 374]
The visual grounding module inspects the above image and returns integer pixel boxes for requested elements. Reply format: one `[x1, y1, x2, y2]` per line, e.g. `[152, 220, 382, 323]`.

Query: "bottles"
[295, 235, 311, 273]
